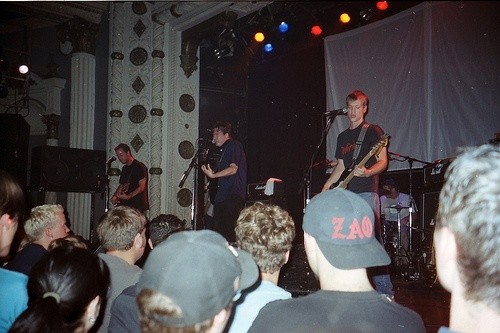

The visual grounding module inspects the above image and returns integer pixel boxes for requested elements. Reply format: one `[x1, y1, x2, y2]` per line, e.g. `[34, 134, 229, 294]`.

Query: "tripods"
[389, 209, 422, 280]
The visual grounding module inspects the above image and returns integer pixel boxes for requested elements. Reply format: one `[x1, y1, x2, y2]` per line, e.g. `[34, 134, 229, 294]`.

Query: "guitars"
[330, 134, 390, 190]
[202, 148, 218, 205]
[116, 180, 129, 207]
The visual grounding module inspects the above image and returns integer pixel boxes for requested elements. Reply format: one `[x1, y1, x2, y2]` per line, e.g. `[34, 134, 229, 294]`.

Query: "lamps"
[215, 37, 242, 59]
[60, 31, 79, 55]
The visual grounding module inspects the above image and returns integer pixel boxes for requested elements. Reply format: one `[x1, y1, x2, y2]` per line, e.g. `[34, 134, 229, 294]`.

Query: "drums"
[381, 213, 385, 226]
[383, 220, 398, 249]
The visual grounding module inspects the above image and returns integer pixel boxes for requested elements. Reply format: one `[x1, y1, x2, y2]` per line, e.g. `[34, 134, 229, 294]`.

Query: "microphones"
[107, 156, 116, 164]
[198, 137, 216, 144]
[324, 108, 349, 116]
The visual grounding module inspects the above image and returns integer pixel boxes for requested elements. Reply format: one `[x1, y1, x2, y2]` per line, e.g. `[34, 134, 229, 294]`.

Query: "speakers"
[422, 191, 444, 279]
[0, 112, 31, 179]
[30, 145, 106, 193]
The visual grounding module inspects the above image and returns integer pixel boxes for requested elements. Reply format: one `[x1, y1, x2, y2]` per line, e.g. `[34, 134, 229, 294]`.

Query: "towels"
[264, 178, 282, 196]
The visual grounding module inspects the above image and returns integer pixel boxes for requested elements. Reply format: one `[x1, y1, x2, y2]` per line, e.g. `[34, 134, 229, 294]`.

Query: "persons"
[433, 143, 500, 333]
[0, 174, 186, 333]
[110, 143, 150, 213]
[322, 91, 394, 302]
[136, 229, 260, 333]
[222, 202, 296, 333]
[247, 186, 427, 333]
[200, 121, 249, 242]
[379, 178, 419, 276]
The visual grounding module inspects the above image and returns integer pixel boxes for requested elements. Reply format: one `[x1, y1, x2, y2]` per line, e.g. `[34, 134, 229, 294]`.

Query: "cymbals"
[385, 204, 409, 209]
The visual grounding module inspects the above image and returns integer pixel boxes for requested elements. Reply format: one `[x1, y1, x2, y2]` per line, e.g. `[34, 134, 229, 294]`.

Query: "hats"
[136, 229, 260, 325]
[302, 188, 392, 270]
